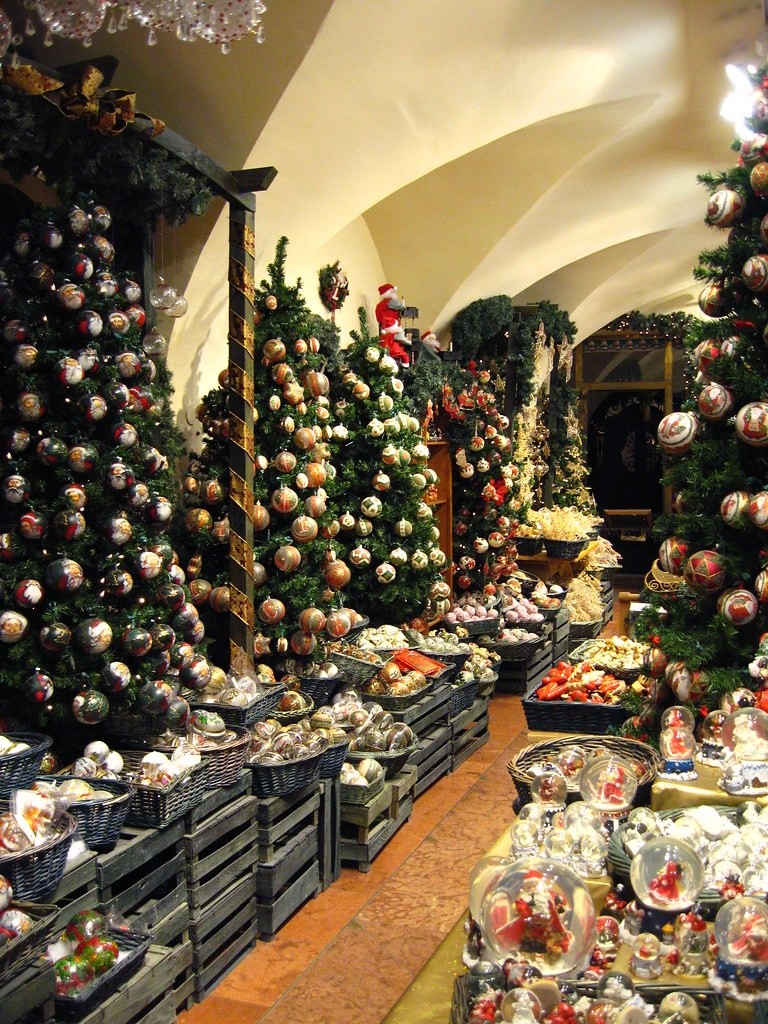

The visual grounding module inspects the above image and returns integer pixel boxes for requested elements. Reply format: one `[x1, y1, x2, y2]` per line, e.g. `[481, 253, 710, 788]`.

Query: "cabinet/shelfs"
[420, 439, 454, 607]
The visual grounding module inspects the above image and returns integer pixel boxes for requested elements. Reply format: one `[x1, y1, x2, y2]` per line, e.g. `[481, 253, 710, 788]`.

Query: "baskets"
[179, 679, 289, 730]
[505, 734, 661, 809]
[0, 900, 63, 987]
[52, 926, 153, 1020]
[315, 740, 350, 778]
[342, 733, 418, 781]
[518, 641, 671, 740]
[265, 688, 316, 726]
[450, 973, 728, 1024]
[241, 744, 328, 798]
[299, 525, 605, 723]
[0, 775, 138, 850]
[605, 806, 768, 920]
[0, 732, 54, 799]
[0, 805, 79, 901]
[333, 764, 388, 805]
[55, 749, 211, 830]
[120, 722, 253, 788]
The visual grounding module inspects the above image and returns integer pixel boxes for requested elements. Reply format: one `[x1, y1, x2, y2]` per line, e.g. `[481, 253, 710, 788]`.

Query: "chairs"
[604, 509, 655, 570]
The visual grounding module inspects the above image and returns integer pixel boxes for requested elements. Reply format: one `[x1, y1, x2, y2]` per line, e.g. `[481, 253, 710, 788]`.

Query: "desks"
[515, 540, 599, 588]
